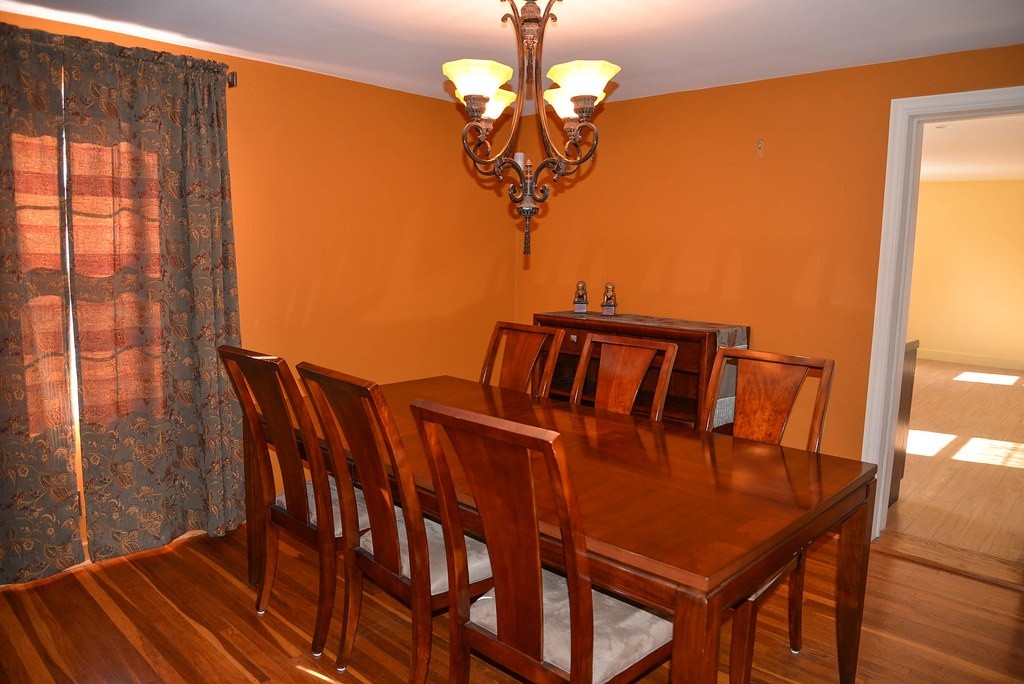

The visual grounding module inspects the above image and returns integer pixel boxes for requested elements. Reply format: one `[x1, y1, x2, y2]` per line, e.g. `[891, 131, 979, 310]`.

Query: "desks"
[241, 375, 878, 684]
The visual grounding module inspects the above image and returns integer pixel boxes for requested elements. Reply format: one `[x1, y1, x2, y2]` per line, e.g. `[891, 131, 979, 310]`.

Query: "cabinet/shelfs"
[531, 311, 751, 436]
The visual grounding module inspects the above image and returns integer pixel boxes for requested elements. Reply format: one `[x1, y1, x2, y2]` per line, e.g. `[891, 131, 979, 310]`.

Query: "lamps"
[442, 0, 622, 255]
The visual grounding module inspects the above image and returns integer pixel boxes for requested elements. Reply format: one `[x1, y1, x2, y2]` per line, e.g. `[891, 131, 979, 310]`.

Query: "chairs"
[481, 320, 566, 397]
[699, 345, 836, 684]
[216, 344, 402, 657]
[294, 361, 494, 684]
[408, 397, 674, 684]
[569, 332, 679, 424]
